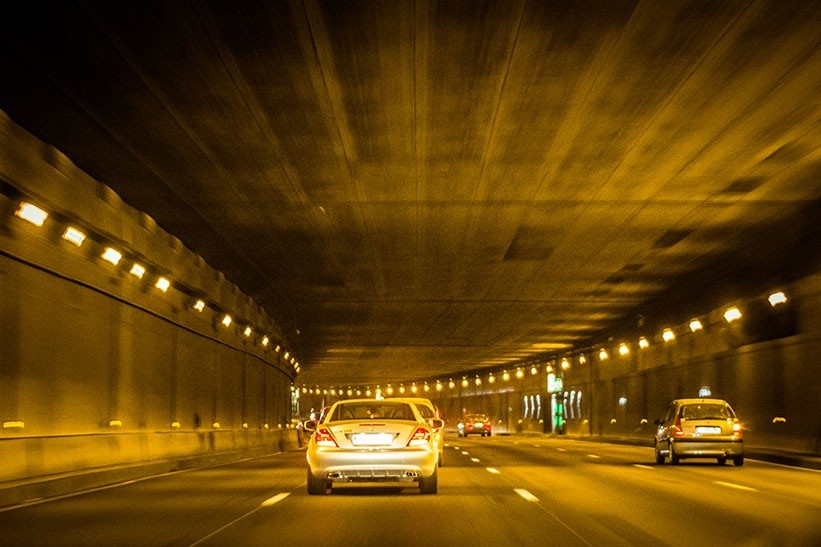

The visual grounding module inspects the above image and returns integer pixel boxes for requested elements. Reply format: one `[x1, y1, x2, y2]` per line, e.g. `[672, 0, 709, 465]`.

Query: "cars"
[302, 398, 444, 494]
[383, 398, 446, 468]
[458, 414, 492, 438]
[654, 399, 744, 467]
